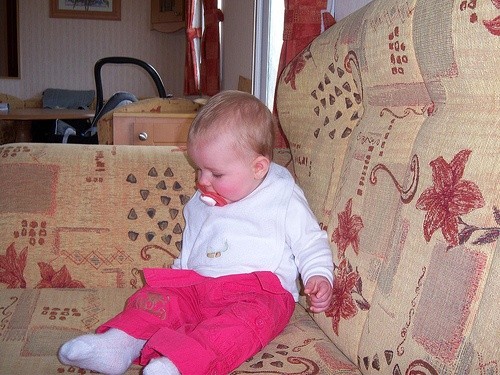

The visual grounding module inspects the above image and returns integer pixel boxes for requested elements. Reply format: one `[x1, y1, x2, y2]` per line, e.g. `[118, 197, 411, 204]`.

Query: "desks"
[0, 107, 96, 142]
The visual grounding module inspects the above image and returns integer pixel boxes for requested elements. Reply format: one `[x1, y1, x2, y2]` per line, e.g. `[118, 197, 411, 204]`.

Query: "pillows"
[40, 88, 95, 110]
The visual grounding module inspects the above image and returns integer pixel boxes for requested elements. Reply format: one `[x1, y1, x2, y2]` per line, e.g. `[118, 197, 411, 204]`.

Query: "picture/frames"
[49, 0, 121, 21]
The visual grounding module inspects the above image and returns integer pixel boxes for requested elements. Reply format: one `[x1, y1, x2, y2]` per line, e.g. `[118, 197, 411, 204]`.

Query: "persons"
[59, 90, 335, 375]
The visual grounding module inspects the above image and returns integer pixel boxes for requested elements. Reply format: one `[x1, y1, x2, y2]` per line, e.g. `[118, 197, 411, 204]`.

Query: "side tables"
[112, 112, 195, 145]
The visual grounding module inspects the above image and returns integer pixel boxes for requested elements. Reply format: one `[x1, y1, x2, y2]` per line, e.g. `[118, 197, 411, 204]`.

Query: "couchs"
[97, 97, 200, 145]
[0, 0, 500, 375]
